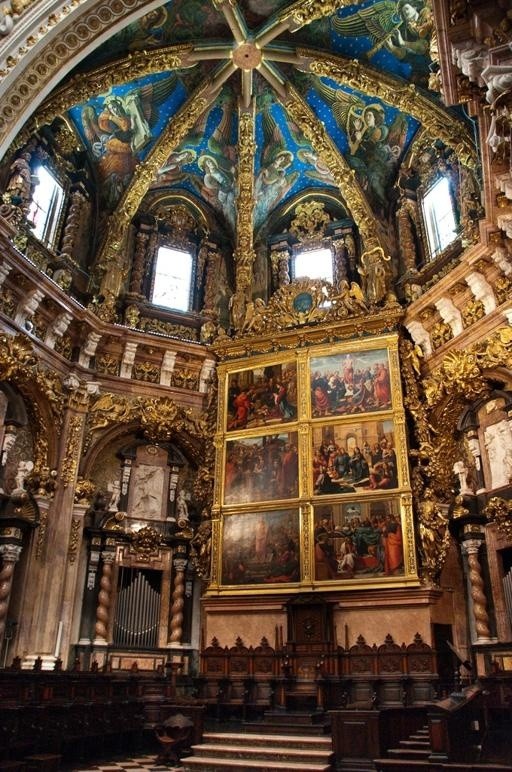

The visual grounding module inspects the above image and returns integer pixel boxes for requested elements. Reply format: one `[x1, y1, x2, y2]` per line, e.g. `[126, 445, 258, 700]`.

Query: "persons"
[225, 389, 403, 582]
[108, 479, 123, 511]
[1, 0, 512, 389]
[14, 460, 34, 493]
[176, 488, 191, 520]
